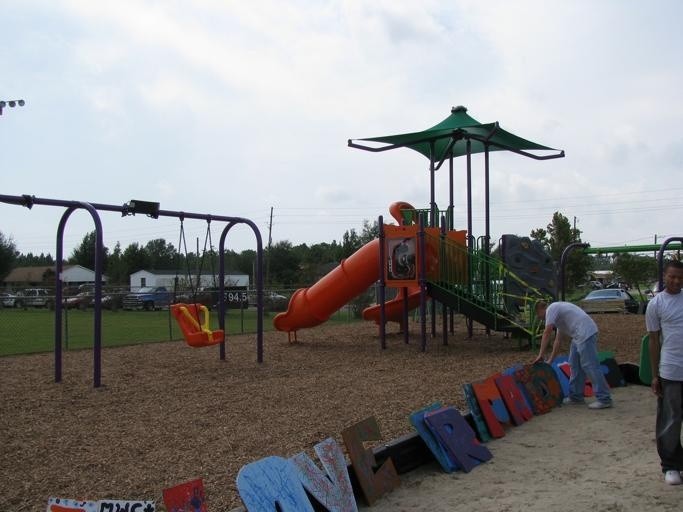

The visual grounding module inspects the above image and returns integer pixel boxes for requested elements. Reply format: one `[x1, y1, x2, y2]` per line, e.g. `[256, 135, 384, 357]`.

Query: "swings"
[171, 212, 225, 349]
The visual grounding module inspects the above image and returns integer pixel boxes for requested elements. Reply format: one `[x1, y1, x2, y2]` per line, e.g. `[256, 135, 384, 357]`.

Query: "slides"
[273, 237, 381, 332]
[362, 282, 427, 325]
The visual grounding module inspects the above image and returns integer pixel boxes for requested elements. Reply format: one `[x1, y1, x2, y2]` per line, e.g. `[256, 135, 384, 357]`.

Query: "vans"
[472, 280, 503, 309]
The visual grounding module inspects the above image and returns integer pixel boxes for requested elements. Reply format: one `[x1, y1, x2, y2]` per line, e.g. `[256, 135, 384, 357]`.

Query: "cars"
[0, 283, 289, 311]
[577, 280, 665, 313]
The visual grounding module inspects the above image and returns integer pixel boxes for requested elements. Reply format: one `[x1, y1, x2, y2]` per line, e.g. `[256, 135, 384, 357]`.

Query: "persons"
[646, 260, 682, 478]
[529, 300, 612, 409]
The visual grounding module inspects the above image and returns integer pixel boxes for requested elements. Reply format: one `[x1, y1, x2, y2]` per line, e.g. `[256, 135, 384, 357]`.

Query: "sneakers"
[588, 401, 612, 409]
[563, 398, 586, 405]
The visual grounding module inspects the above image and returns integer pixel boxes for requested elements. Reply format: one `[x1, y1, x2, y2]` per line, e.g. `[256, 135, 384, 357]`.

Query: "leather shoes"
[665, 470, 681, 484]
